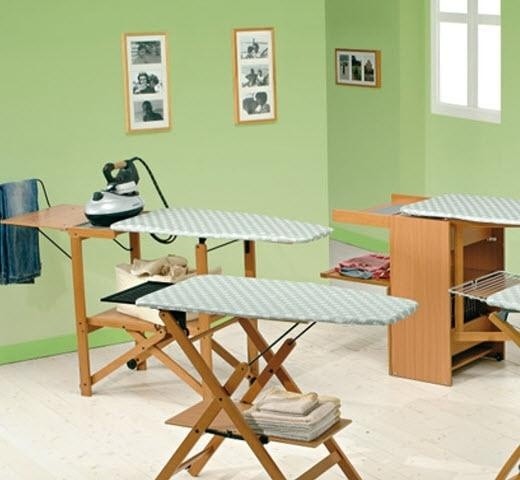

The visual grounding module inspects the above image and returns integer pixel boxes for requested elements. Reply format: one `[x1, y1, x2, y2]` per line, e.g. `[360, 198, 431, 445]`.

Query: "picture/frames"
[233, 28, 277, 125]
[334, 48, 382, 89]
[121, 31, 173, 135]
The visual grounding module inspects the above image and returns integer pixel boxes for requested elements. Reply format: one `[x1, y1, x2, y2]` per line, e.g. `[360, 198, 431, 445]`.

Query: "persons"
[256, 70, 269, 86]
[241, 67, 258, 88]
[134, 74, 160, 93]
[142, 101, 163, 122]
[243, 98, 259, 115]
[252, 43, 262, 57]
[242, 45, 255, 58]
[134, 72, 155, 94]
[133, 43, 152, 64]
[254, 92, 270, 113]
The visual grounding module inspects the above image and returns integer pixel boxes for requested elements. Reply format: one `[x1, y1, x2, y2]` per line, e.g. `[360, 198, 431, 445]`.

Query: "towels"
[0, 179, 42, 285]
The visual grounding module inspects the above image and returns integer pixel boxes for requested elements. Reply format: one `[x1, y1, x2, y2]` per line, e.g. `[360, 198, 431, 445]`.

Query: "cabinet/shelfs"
[334, 194, 520, 387]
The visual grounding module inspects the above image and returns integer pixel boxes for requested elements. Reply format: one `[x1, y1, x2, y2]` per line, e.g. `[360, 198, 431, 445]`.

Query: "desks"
[1, 204, 334, 401]
[448, 272, 520, 480]
[136, 274, 419, 480]
